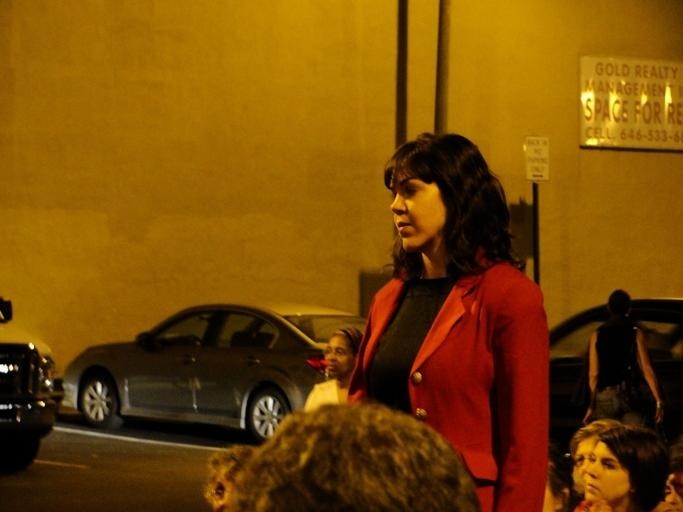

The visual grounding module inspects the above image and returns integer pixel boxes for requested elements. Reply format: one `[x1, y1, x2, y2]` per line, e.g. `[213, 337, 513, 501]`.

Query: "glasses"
[574, 454, 591, 465]
[326, 346, 351, 355]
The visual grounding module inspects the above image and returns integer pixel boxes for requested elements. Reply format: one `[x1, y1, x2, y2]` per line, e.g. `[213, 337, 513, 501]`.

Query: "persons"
[306, 327, 361, 412]
[574, 428, 676, 512]
[581, 289, 664, 429]
[218, 403, 478, 511]
[545, 438, 573, 511]
[664, 441, 682, 510]
[573, 419, 624, 507]
[349, 134, 549, 510]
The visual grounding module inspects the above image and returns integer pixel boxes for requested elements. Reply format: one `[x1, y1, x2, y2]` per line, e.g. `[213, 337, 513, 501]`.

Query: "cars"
[0, 295, 65, 469]
[61, 302, 369, 444]
[549, 298, 683, 440]
